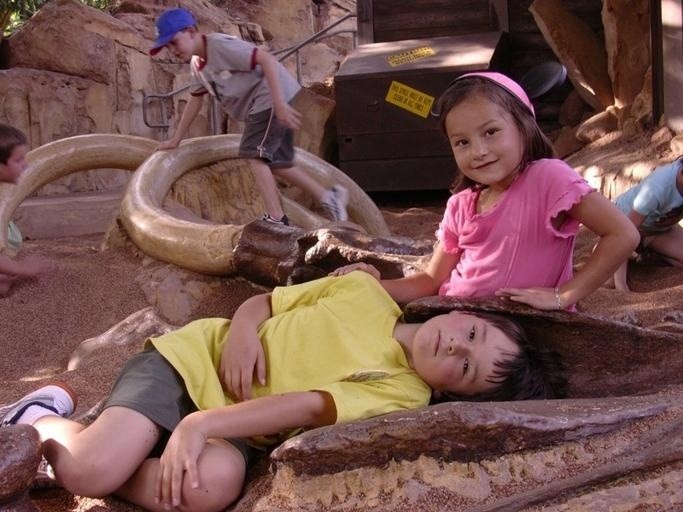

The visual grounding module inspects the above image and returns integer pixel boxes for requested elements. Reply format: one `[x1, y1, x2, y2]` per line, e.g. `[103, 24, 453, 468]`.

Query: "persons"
[605, 153, 683, 294]
[0, 123, 59, 298]
[326, 73, 644, 315]
[155, 10, 351, 228]
[0, 268, 571, 510]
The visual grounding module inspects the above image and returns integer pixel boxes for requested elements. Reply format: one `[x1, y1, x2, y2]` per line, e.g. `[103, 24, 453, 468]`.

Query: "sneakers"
[0, 381, 78, 427]
[319, 184, 349, 222]
[34, 460, 57, 489]
[261, 213, 289, 225]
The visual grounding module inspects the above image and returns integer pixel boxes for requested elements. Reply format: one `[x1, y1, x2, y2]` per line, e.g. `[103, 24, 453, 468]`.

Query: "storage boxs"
[328, 32, 513, 203]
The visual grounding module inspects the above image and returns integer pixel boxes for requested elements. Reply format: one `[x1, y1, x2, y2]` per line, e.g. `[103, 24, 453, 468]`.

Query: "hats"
[149, 7, 198, 56]
[446, 70, 534, 115]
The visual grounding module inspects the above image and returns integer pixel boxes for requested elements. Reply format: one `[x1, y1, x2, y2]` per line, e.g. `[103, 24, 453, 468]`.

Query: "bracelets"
[548, 282, 564, 310]
